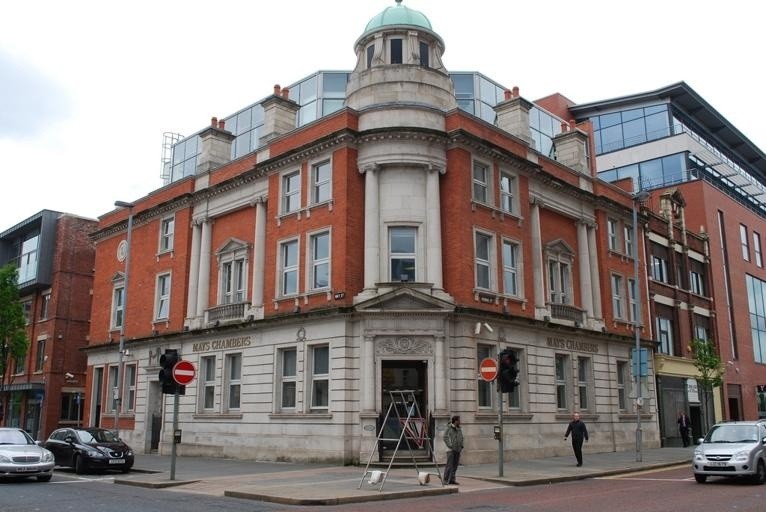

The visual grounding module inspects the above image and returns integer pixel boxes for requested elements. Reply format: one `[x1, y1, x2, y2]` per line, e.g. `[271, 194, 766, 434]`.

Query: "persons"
[677, 410, 692, 447]
[441, 415, 465, 485]
[564, 412, 590, 467]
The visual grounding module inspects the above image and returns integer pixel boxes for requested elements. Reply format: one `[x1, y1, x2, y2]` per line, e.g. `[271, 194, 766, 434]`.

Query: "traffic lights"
[498, 350, 520, 395]
[158, 349, 179, 395]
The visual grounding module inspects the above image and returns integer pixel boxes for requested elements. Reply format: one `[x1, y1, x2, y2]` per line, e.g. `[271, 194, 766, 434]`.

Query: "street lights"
[630, 188, 651, 465]
[111, 199, 136, 439]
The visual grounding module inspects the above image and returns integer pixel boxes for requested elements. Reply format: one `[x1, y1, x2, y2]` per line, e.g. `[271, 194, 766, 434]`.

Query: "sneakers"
[442, 480, 460, 485]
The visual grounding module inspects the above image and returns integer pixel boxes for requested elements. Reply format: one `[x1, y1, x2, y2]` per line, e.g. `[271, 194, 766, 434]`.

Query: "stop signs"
[172, 361, 195, 385]
[479, 356, 499, 383]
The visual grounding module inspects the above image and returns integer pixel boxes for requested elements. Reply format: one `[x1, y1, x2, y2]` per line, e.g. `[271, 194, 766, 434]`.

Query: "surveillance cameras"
[483, 322, 493, 332]
[475, 322, 481, 335]
[65, 373, 74, 379]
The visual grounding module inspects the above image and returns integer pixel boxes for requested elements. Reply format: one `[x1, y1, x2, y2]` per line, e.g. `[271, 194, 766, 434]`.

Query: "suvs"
[690, 418, 766, 483]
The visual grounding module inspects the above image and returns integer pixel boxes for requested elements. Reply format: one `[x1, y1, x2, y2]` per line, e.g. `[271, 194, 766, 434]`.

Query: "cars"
[46, 426, 135, 475]
[0, 428, 55, 483]
